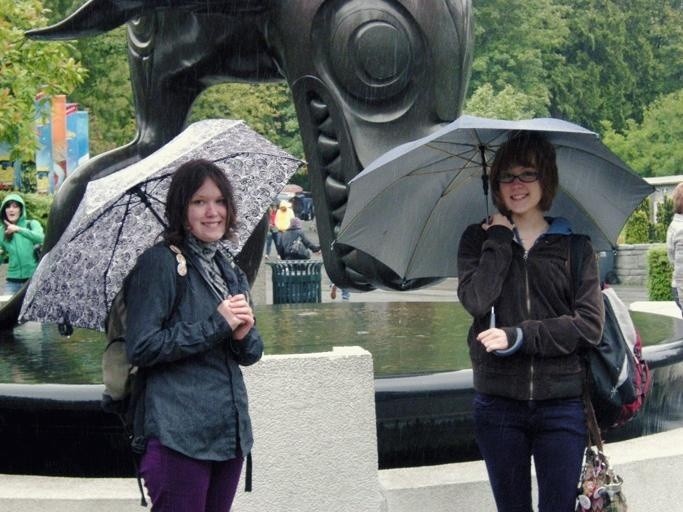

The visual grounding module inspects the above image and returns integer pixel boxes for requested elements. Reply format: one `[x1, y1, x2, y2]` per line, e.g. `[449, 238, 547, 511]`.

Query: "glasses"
[496, 172, 539, 183]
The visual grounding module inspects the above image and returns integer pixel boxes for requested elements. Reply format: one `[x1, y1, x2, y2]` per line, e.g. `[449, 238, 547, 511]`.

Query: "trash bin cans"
[264, 259, 325, 303]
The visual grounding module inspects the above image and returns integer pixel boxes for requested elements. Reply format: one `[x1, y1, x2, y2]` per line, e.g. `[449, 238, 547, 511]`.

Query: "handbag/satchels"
[575, 445, 628, 512]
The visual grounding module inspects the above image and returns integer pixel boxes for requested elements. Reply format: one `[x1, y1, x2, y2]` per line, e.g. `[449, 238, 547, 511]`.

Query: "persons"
[0, 195, 45, 294]
[127, 161, 262, 512]
[667, 183, 682, 315]
[458, 136, 605, 512]
[265, 200, 320, 259]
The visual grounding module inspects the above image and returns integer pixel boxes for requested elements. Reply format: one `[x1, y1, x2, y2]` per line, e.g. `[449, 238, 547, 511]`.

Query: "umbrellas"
[18, 119, 307, 332]
[331, 115, 656, 357]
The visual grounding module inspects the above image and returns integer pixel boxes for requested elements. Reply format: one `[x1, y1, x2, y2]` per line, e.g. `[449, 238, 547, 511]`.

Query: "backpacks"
[569, 233, 650, 427]
[102, 241, 188, 412]
